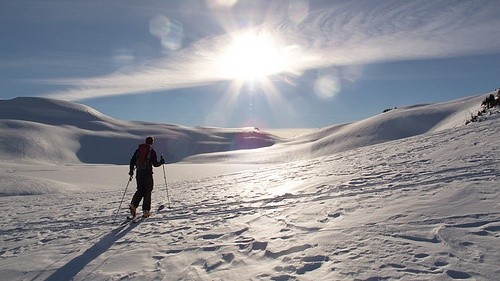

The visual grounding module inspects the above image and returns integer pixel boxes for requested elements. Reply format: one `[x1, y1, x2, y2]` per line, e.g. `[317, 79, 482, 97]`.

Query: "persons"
[129, 136, 165, 217]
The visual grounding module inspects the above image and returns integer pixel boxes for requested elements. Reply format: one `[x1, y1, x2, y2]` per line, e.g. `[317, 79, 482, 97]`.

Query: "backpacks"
[136, 144, 152, 169]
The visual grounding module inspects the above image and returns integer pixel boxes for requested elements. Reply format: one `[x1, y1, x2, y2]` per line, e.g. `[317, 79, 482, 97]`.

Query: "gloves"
[129, 172, 133, 175]
[160, 159, 165, 164]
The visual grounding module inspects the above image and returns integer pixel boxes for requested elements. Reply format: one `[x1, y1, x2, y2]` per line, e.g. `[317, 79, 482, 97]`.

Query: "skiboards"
[126, 204, 164, 223]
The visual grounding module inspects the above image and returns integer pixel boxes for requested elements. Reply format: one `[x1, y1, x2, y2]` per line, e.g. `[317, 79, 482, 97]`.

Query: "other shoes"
[143, 212, 150, 217]
[130, 206, 136, 217]
